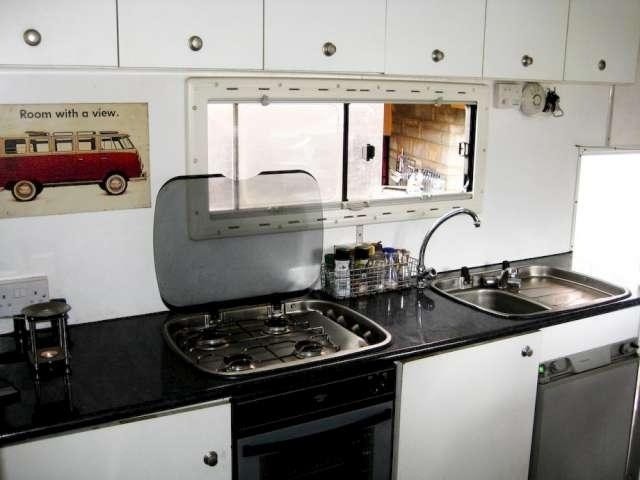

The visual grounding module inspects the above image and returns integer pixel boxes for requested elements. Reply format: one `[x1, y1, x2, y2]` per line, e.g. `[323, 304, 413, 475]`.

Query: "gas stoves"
[164, 294, 393, 380]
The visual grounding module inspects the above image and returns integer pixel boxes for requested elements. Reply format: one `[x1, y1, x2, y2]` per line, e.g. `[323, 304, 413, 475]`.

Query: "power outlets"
[0, 276, 50, 320]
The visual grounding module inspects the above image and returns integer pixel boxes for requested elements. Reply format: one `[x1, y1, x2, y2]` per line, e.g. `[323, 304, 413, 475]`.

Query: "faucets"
[416, 287, 435, 344]
[415, 206, 481, 289]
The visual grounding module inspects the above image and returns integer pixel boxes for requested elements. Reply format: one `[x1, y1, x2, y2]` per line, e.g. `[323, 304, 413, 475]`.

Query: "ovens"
[231, 361, 397, 479]
[527, 335, 640, 479]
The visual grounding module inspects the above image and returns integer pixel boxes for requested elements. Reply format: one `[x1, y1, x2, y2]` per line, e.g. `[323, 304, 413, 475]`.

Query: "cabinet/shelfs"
[481, 0, 640, 83]
[0, 399, 233, 480]
[261, 2, 485, 81]
[2, 1, 264, 84]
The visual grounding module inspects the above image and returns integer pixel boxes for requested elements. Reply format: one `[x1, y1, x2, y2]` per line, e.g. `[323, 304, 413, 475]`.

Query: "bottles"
[324, 242, 411, 302]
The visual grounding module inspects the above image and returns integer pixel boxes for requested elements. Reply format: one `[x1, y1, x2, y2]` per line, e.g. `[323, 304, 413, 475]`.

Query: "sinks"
[446, 286, 553, 318]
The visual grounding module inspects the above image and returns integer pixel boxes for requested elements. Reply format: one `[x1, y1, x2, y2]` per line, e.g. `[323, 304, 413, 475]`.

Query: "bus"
[0, 128, 146, 203]
